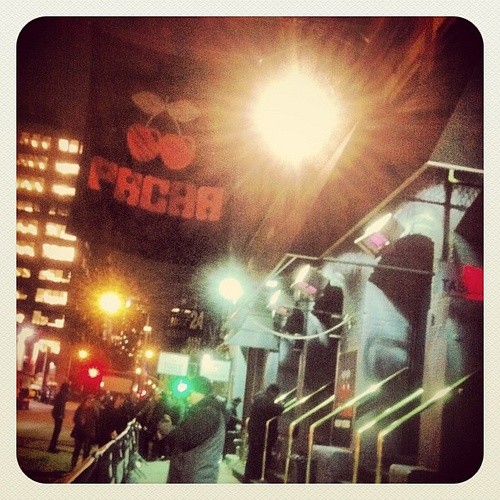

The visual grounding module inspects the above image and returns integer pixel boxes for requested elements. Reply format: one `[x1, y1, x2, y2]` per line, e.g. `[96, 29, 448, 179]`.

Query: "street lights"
[66, 349, 88, 385]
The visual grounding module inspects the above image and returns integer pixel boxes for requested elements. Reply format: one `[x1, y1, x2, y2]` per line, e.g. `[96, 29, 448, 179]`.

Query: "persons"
[70, 387, 178, 471]
[47, 382, 69, 452]
[155, 376, 225, 484]
[240, 385, 288, 484]
[224, 397, 244, 455]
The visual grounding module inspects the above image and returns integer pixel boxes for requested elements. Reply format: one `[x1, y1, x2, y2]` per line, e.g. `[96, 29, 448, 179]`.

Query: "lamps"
[353, 213, 405, 259]
[288, 264, 323, 296]
